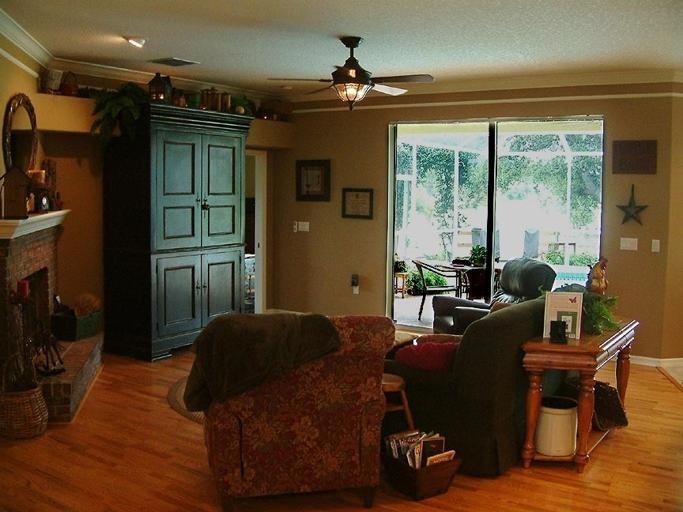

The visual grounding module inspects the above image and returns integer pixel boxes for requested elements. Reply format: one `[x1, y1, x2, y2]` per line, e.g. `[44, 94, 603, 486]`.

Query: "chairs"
[432, 258, 556, 336]
[197, 311, 395, 511]
[412, 258, 501, 322]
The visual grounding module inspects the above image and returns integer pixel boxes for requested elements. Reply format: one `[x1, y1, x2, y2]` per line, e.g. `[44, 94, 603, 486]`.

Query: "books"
[543, 288, 582, 341]
[386, 429, 454, 472]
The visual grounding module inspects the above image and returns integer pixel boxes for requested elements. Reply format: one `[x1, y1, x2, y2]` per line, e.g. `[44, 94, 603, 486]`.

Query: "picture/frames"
[294, 158, 373, 220]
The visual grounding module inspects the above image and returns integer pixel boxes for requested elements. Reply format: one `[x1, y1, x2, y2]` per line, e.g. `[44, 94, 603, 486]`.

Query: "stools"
[381, 371, 418, 441]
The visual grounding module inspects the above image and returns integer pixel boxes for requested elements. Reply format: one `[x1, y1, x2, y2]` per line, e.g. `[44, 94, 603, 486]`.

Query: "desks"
[521, 311, 639, 472]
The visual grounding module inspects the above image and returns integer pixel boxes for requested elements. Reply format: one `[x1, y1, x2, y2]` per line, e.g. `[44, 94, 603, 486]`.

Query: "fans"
[265, 36, 435, 97]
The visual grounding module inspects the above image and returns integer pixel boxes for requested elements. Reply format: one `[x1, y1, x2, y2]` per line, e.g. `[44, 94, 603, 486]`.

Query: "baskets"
[0, 352, 48, 438]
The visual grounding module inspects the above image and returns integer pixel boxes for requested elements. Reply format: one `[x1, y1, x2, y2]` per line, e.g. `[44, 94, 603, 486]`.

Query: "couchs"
[378, 284, 587, 478]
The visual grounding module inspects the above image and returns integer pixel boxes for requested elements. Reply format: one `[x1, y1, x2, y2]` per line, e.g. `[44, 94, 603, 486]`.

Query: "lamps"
[333, 79, 374, 111]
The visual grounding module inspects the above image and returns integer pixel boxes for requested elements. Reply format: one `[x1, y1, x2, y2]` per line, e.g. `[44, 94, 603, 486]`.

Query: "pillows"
[394, 331, 462, 372]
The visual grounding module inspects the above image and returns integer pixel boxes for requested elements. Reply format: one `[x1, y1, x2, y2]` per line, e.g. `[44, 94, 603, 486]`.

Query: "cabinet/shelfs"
[98, 102, 256, 363]
